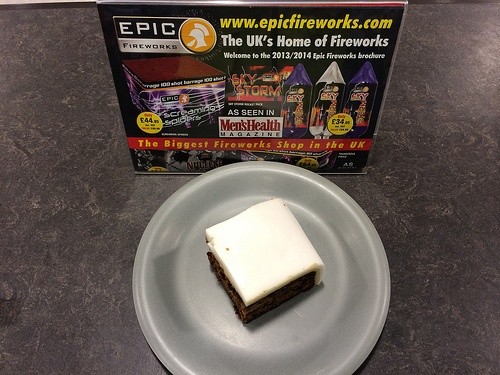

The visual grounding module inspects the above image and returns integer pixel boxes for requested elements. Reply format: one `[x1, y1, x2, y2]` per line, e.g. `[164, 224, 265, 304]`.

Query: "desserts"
[204, 198, 326, 326]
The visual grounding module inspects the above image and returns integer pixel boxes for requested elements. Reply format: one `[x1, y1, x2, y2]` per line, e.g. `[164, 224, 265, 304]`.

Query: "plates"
[130, 157, 390, 374]
